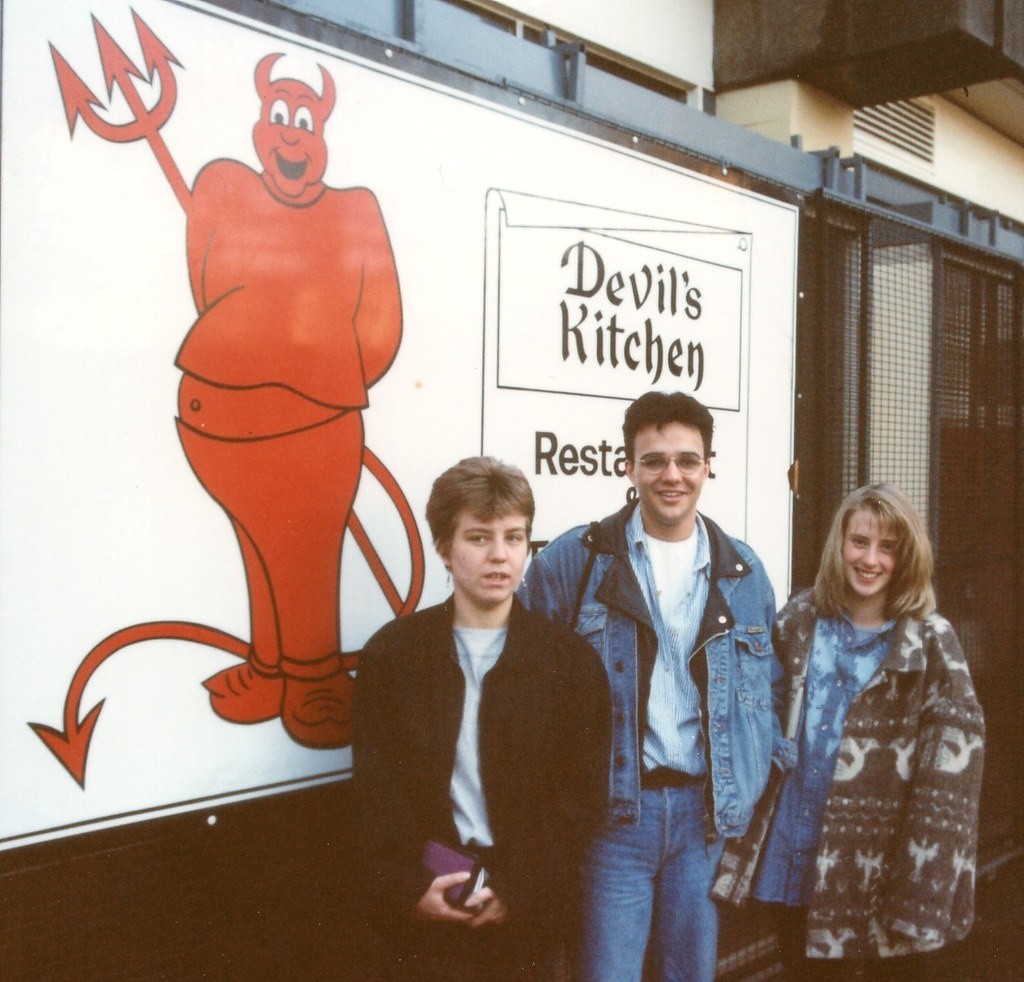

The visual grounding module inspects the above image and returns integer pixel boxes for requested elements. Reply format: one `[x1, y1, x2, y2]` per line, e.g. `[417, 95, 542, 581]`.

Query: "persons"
[708, 483, 985, 982]
[350, 456, 611, 982]
[516, 391, 798, 982]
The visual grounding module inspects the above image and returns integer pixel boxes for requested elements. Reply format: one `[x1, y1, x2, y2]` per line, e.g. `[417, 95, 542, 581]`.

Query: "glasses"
[631, 451, 708, 475]
